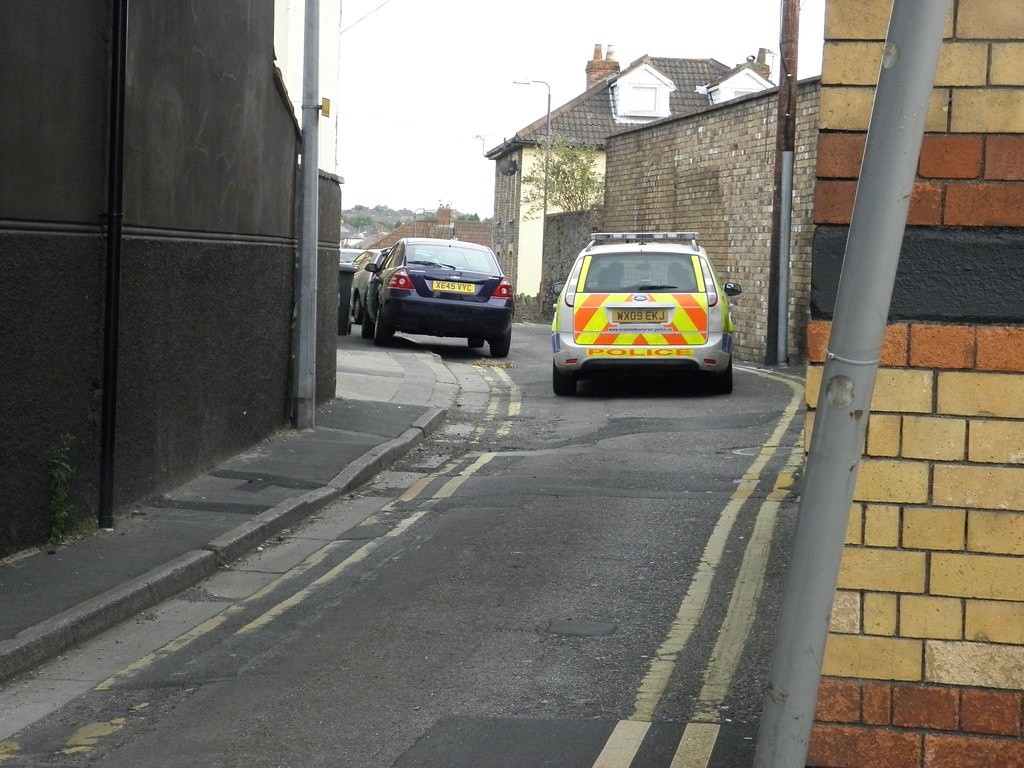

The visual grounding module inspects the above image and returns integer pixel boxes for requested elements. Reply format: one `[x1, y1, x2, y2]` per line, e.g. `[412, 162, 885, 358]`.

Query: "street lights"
[511, 80, 552, 323]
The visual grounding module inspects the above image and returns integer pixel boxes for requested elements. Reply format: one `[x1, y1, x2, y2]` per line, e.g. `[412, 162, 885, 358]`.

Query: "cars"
[550, 231, 743, 395]
[338, 237, 515, 357]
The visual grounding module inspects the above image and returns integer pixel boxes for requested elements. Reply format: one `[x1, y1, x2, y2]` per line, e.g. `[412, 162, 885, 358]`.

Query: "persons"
[665, 262, 684, 285]
[606, 261, 633, 290]
